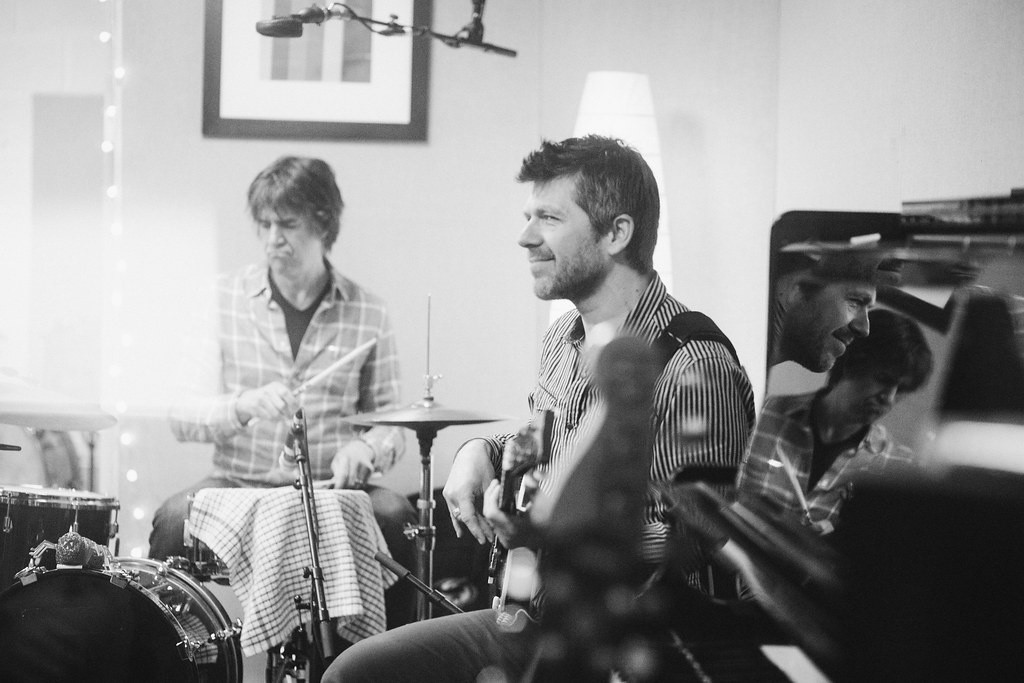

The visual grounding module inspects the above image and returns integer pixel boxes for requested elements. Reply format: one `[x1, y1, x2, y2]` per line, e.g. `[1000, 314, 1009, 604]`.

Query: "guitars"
[481, 317, 664, 647]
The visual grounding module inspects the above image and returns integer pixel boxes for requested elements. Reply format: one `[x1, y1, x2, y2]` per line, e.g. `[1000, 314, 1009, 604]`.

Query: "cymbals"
[341, 404, 505, 432]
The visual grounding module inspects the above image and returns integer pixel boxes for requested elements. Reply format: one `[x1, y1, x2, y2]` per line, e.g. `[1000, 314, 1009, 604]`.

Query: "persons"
[320, 134, 756, 683]
[737, 267, 937, 534]
[143, 155, 418, 633]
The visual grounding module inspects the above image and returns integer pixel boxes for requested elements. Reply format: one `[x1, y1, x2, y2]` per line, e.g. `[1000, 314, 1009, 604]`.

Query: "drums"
[1, 557, 246, 683]
[0, 481, 122, 583]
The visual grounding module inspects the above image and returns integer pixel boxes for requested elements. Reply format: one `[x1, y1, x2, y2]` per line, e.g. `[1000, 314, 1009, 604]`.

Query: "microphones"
[256, 13, 304, 38]
[279, 407, 305, 474]
